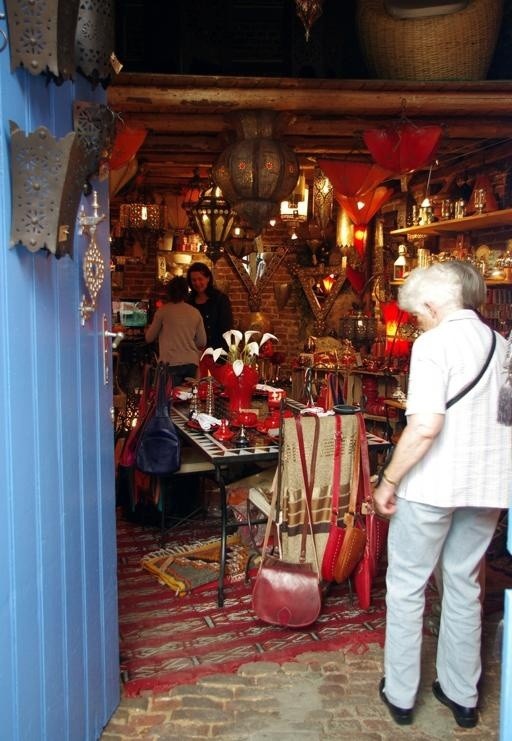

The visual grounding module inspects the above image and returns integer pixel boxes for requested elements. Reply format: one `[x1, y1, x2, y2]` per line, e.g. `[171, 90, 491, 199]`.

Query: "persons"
[145, 276, 207, 386]
[187, 262, 232, 361]
[443, 261, 489, 615]
[371, 265, 511, 727]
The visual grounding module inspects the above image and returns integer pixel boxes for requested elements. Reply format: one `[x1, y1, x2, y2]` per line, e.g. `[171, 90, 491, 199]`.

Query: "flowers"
[198, 329, 282, 379]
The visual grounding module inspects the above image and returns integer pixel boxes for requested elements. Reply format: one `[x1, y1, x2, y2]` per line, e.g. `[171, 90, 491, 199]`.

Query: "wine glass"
[471, 188, 487, 216]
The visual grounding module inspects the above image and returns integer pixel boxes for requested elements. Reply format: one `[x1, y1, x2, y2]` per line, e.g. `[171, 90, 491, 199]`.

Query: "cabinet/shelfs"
[296, 362, 405, 435]
[386, 207, 512, 288]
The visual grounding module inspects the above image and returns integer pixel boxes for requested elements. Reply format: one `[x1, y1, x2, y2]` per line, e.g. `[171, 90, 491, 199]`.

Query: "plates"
[332, 405, 361, 415]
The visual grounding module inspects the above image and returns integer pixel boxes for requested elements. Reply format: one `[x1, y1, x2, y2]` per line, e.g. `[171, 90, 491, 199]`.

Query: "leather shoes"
[376, 672, 415, 726]
[430, 678, 481, 729]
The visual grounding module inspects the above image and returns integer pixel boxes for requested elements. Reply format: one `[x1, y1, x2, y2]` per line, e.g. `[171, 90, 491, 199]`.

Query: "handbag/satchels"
[322, 521, 343, 583]
[262, 555, 313, 575]
[351, 509, 390, 615]
[339, 524, 364, 581]
[119, 363, 182, 475]
[253, 567, 320, 632]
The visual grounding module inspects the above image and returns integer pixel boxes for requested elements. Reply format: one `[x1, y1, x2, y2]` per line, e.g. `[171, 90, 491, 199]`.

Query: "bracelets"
[384, 474, 403, 493]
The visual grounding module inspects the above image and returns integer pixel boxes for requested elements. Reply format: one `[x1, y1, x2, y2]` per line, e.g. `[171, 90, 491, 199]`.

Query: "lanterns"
[110, 160, 138, 197]
[216, 113, 300, 232]
[363, 127, 442, 176]
[318, 159, 392, 197]
[110, 121, 147, 170]
[335, 186, 393, 226]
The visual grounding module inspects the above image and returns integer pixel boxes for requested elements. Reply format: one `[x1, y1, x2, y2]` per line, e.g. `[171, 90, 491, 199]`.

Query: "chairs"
[242, 408, 362, 607]
[139, 362, 225, 550]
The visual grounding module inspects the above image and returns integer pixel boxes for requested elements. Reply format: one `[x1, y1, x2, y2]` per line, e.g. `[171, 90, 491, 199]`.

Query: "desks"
[165, 385, 396, 612]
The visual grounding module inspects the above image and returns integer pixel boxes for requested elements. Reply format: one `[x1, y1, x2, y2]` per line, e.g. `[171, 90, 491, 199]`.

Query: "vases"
[217, 363, 262, 430]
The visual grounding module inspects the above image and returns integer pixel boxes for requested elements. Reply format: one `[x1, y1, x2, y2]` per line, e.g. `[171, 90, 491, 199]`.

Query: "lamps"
[76, 189, 107, 326]
[113, 107, 448, 264]
[337, 302, 378, 351]
[296, 1, 323, 43]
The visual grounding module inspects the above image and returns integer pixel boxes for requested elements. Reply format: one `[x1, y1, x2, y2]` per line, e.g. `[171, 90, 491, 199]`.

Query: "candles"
[267, 393, 283, 412]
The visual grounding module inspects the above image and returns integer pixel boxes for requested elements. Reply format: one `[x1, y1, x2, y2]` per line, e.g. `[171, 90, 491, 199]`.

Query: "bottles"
[306, 394, 315, 416]
[440, 197, 466, 221]
[189, 386, 201, 415]
[303, 337, 316, 354]
[393, 244, 411, 281]
[213, 405, 299, 446]
[417, 207, 434, 226]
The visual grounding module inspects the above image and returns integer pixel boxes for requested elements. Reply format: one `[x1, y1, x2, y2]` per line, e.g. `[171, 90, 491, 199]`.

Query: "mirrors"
[287, 265, 353, 335]
[223, 242, 292, 313]
